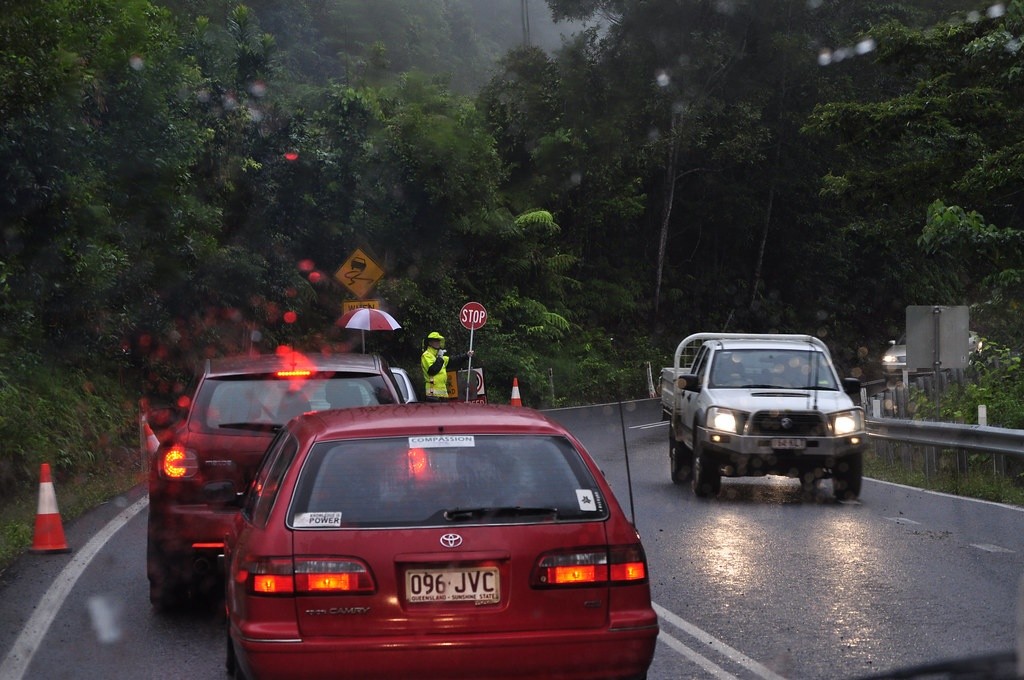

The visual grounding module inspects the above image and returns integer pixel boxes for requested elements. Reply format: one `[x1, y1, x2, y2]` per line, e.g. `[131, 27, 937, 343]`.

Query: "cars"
[880, 330, 982, 378]
[221, 398, 661, 680]
[309, 365, 418, 415]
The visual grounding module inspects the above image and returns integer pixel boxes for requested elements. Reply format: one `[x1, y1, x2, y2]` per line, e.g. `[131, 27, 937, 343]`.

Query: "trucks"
[655, 331, 868, 508]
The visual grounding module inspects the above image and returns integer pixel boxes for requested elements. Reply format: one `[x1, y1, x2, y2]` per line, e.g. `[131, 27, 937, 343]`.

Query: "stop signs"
[458, 302, 487, 330]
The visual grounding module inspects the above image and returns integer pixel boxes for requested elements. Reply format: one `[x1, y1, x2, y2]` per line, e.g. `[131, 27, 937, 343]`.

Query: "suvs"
[141, 344, 433, 610]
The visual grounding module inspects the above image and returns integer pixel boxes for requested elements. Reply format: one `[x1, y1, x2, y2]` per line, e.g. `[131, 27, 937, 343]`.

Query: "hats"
[428, 332, 444, 339]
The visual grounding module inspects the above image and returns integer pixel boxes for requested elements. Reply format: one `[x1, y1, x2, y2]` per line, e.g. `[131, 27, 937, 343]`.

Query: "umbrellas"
[334, 308, 401, 331]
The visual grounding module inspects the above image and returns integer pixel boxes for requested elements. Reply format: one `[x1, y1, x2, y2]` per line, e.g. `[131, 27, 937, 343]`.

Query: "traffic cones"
[144, 421, 161, 455]
[510, 377, 522, 406]
[28, 460, 70, 556]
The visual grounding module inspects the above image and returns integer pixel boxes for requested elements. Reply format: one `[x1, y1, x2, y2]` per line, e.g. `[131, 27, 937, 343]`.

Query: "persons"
[276, 391, 312, 423]
[420, 332, 474, 401]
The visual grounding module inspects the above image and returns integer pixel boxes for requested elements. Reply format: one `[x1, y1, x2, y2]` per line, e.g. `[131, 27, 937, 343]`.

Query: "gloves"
[467, 351, 474, 356]
[438, 350, 443, 356]
